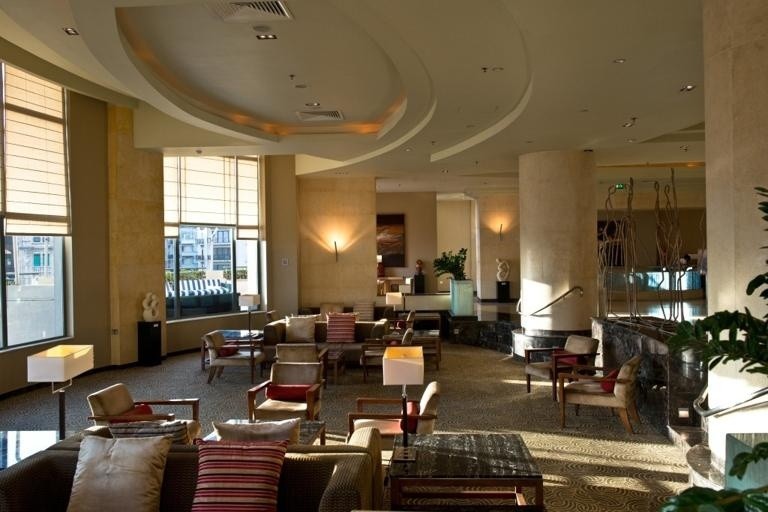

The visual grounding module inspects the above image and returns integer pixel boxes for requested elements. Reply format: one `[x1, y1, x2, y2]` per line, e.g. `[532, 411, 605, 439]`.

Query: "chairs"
[199, 308, 419, 421]
[86, 382, 200, 440]
[525, 335, 643, 435]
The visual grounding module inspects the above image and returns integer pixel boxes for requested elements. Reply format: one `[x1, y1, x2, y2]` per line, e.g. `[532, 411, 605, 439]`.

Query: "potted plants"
[432, 247, 477, 316]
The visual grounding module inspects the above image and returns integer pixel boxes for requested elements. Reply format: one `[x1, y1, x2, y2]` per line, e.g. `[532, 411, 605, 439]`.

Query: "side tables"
[391, 432, 544, 511]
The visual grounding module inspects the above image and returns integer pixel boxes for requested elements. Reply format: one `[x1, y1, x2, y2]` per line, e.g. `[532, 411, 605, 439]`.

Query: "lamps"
[385, 291, 402, 316]
[382, 344, 424, 460]
[26, 344, 94, 440]
